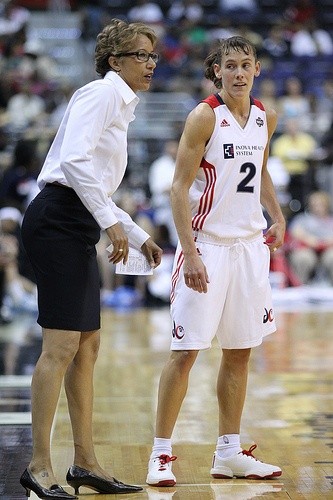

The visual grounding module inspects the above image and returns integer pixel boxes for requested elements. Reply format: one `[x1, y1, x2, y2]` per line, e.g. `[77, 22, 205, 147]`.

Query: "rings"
[273, 248, 278, 252]
[118, 249, 125, 251]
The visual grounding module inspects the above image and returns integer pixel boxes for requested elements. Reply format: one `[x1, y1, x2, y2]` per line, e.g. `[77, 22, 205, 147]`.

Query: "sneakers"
[148, 454, 180, 487]
[210, 444, 279, 481]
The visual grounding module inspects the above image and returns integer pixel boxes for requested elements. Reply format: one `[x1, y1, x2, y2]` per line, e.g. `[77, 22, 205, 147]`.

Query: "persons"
[143, 36, 286, 486]
[0, 0, 333, 375]
[20, 18, 163, 500]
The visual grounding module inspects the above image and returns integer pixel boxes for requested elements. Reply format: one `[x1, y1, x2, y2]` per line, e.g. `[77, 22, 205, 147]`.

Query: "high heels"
[19, 467, 142, 500]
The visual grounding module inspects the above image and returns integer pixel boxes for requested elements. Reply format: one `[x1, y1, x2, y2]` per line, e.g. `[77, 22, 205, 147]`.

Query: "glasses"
[112, 50, 160, 62]
